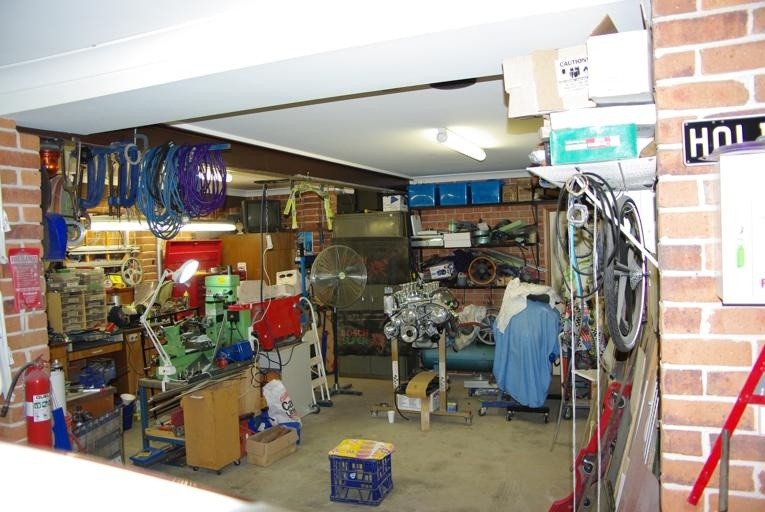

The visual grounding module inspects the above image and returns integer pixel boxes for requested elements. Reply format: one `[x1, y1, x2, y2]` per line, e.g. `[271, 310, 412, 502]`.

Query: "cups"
[387, 410, 396, 424]
[120, 393, 137, 405]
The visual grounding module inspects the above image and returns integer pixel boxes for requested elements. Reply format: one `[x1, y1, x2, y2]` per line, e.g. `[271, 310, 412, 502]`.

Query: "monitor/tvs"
[241, 199, 281, 233]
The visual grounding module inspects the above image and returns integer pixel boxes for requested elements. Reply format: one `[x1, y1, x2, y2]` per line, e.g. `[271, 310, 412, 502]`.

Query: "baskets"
[327, 453, 396, 507]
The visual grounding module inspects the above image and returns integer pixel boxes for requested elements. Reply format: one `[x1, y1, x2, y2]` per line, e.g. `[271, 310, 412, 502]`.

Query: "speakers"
[336, 193, 355, 212]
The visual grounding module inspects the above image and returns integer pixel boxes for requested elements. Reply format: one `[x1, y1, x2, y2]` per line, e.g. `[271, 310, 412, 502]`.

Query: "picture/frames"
[540, 199, 606, 385]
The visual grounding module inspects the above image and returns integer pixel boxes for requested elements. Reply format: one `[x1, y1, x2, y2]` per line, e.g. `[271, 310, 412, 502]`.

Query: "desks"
[134, 353, 262, 455]
[48, 321, 171, 420]
[62, 383, 118, 425]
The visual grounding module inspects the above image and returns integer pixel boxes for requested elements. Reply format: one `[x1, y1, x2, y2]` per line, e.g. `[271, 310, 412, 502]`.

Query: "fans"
[308, 243, 370, 400]
[466, 255, 498, 286]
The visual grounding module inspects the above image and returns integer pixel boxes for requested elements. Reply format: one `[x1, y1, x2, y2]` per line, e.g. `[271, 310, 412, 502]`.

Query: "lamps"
[85, 210, 238, 234]
[38, 135, 69, 180]
[432, 126, 487, 163]
[137, 258, 202, 394]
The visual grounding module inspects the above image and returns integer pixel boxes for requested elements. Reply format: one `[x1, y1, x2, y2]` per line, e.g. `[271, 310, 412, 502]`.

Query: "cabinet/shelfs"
[179, 380, 243, 477]
[326, 211, 420, 382]
[215, 231, 297, 286]
[254, 342, 316, 421]
[408, 198, 542, 292]
[45, 286, 110, 336]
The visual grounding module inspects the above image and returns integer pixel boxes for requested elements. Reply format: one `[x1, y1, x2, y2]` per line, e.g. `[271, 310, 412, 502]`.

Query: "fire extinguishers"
[1, 353, 55, 448]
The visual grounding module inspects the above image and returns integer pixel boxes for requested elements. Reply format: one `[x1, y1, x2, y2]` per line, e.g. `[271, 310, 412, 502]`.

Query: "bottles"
[447, 216, 458, 235]
[49, 358, 67, 417]
[110, 293, 121, 306]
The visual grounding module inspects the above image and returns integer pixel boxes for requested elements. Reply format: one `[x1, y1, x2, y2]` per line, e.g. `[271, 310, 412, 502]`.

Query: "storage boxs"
[466, 177, 506, 206]
[421, 262, 451, 280]
[333, 188, 407, 214]
[406, 183, 439, 209]
[273, 266, 310, 295]
[245, 422, 300, 469]
[395, 388, 440, 415]
[498, 13, 657, 166]
[436, 180, 472, 207]
[440, 230, 474, 250]
